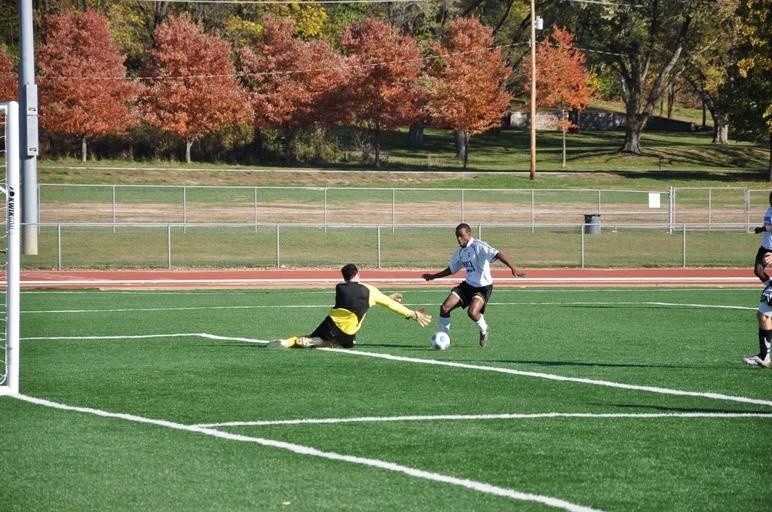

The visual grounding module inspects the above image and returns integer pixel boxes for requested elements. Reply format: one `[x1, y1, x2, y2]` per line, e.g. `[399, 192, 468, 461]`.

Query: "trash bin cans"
[583, 213, 602, 234]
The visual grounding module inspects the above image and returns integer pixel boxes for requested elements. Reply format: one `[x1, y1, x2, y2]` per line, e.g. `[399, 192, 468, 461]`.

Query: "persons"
[741, 249, 771, 369]
[751, 192, 771, 287]
[265, 264, 433, 350]
[421, 223, 528, 348]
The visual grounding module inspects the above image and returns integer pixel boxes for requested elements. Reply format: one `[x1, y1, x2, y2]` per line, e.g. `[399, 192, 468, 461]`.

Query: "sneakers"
[480, 326, 489, 348]
[742, 357, 771, 368]
[265, 335, 322, 349]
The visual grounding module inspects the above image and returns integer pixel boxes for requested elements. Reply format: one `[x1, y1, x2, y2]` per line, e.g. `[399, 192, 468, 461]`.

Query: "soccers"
[431, 332, 450, 350]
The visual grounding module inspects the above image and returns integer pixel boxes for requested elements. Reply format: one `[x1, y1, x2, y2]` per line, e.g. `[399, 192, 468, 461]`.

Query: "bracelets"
[414, 310, 419, 319]
